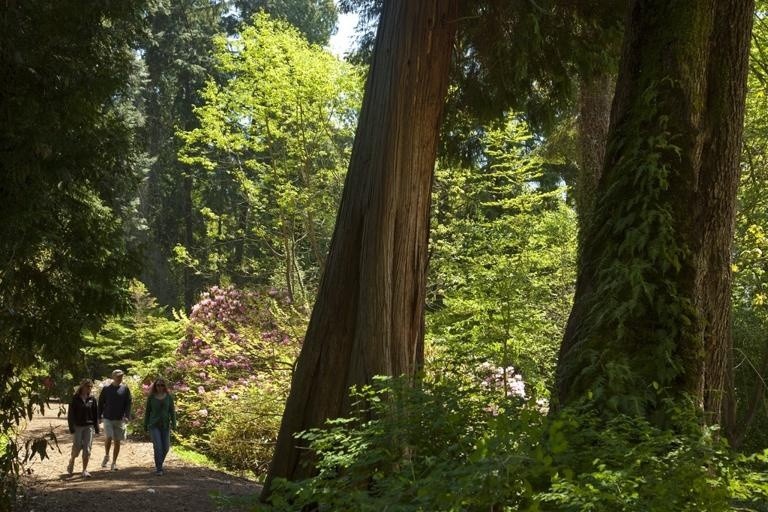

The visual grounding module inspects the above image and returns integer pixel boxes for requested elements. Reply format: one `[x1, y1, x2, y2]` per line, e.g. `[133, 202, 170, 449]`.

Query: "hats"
[111, 369, 125, 377]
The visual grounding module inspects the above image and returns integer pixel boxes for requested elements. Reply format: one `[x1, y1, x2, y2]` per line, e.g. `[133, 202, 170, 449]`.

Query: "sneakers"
[81, 470, 91, 477]
[155, 470, 164, 476]
[111, 462, 116, 472]
[67, 465, 74, 475]
[102, 458, 108, 467]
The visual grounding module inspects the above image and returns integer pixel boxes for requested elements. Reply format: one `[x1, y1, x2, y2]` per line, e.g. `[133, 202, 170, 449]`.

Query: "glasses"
[157, 383, 165, 387]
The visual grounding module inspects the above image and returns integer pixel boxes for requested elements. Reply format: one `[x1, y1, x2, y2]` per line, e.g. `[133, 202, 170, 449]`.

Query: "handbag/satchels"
[143, 430, 151, 441]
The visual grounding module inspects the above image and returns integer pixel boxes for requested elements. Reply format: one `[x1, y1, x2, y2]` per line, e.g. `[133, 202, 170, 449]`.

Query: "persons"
[98, 370, 132, 471]
[66, 378, 100, 478]
[142, 378, 178, 477]
